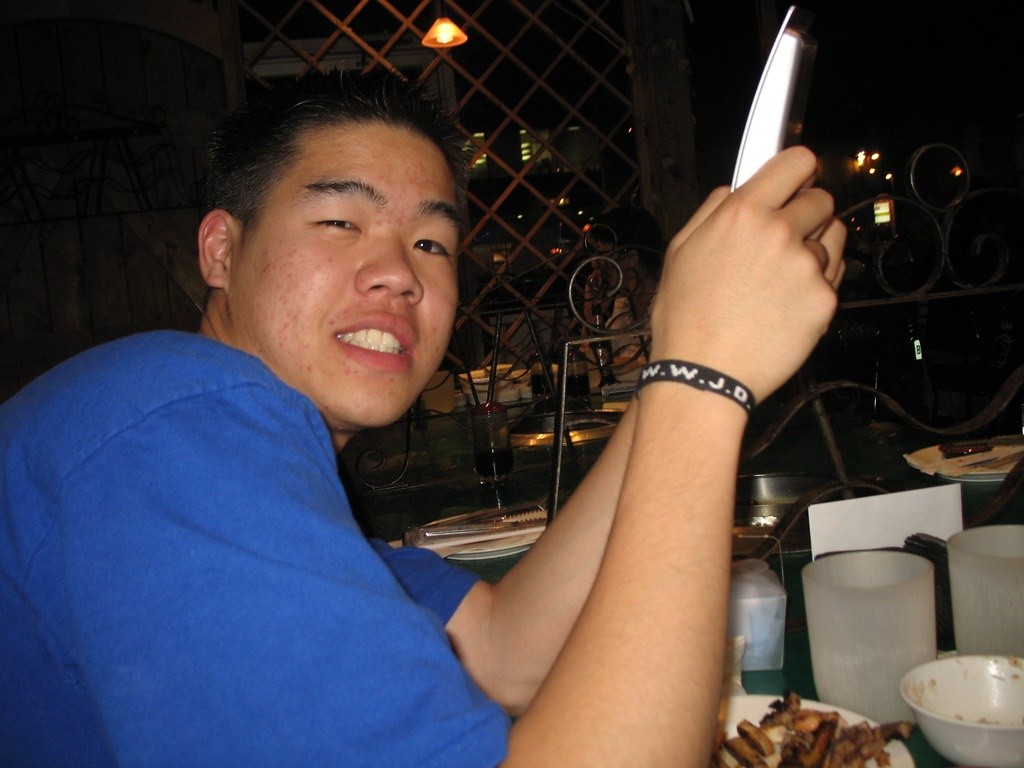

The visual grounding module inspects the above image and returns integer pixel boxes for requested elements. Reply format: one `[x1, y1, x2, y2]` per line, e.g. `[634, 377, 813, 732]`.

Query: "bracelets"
[635, 358, 755, 419]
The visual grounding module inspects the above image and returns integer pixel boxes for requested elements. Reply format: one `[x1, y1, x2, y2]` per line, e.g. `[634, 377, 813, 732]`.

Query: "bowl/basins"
[420, 366, 532, 414]
[900, 654, 1024, 768]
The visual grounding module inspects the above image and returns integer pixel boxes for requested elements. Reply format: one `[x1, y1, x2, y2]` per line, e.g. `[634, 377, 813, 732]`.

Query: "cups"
[470, 408, 513, 488]
[947, 525, 1024, 658]
[801, 550, 937, 726]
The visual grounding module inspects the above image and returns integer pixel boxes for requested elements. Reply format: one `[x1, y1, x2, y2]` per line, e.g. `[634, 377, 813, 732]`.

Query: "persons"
[579, 207, 667, 363]
[0, 70, 848, 767]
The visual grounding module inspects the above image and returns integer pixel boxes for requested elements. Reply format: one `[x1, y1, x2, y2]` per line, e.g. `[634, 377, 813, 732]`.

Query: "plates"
[714, 695, 915, 768]
[387, 539, 532, 562]
[935, 471, 1007, 482]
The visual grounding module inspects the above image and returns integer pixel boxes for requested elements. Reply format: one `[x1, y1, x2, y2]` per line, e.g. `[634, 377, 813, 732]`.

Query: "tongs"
[402, 499, 551, 546]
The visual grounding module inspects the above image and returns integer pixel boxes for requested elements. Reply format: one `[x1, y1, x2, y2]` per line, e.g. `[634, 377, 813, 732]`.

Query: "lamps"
[421, 0, 469, 49]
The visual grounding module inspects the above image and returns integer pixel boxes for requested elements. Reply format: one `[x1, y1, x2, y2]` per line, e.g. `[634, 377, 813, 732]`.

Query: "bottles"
[565, 341, 591, 410]
[530, 344, 555, 412]
[591, 303, 620, 386]
[728, 561, 787, 673]
